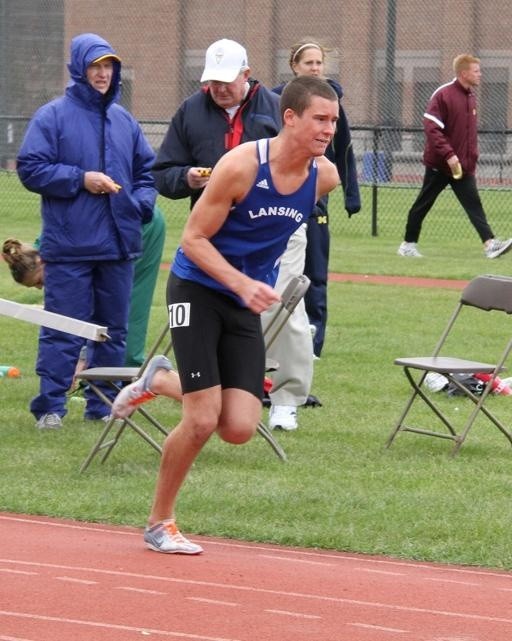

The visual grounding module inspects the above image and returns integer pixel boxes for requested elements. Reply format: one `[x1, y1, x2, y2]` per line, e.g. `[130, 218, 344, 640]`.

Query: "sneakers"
[110, 356, 172, 418]
[266, 356, 280, 371]
[484, 237, 512, 258]
[271, 406, 299, 432]
[396, 240, 420, 257]
[143, 517, 205, 555]
[35, 411, 63, 431]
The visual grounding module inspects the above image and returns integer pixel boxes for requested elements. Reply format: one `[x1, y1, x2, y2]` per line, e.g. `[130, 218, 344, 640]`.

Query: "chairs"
[385, 274, 512, 458]
[76, 324, 172, 475]
[257, 276, 310, 461]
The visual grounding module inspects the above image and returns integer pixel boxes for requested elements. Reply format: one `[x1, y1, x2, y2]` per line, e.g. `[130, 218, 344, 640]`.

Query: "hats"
[199, 38, 249, 84]
[92, 55, 121, 65]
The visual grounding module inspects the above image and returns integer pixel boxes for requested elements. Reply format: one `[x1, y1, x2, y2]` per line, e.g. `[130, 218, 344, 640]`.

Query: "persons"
[272, 35, 364, 360]
[394, 52, 511, 264]
[109, 73, 345, 560]
[0, 198, 167, 388]
[153, 35, 324, 433]
[11, 34, 158, 425]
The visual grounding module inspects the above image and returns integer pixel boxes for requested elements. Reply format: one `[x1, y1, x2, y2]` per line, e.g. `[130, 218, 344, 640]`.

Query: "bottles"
[0, 366, 19, 378]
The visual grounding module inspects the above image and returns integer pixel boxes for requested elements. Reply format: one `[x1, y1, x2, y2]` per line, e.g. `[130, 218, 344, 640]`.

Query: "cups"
[472, 371, 512, 398]
